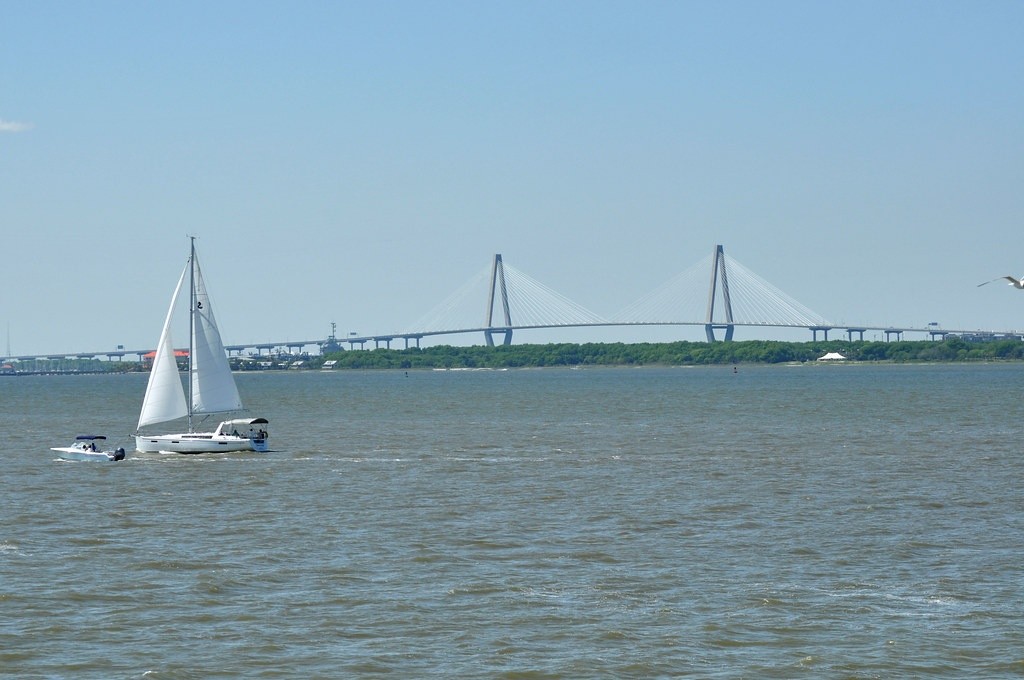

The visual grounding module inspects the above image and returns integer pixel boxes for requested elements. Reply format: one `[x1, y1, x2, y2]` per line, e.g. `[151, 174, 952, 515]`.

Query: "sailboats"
[128, 234, 279, 455]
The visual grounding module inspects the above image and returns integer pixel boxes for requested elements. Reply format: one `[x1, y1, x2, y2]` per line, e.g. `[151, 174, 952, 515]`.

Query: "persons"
[232, 430, 239, 437]
[89, 443, 96, 452]
[259, 429, 264, 440]
[249, 429, 254, 439]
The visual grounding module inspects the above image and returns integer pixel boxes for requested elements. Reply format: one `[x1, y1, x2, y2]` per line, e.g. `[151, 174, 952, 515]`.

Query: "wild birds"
[977, 276, 1024, 289]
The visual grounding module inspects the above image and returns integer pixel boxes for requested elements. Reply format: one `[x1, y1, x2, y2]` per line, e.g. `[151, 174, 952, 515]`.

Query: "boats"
[50, 435, 125, 463]
